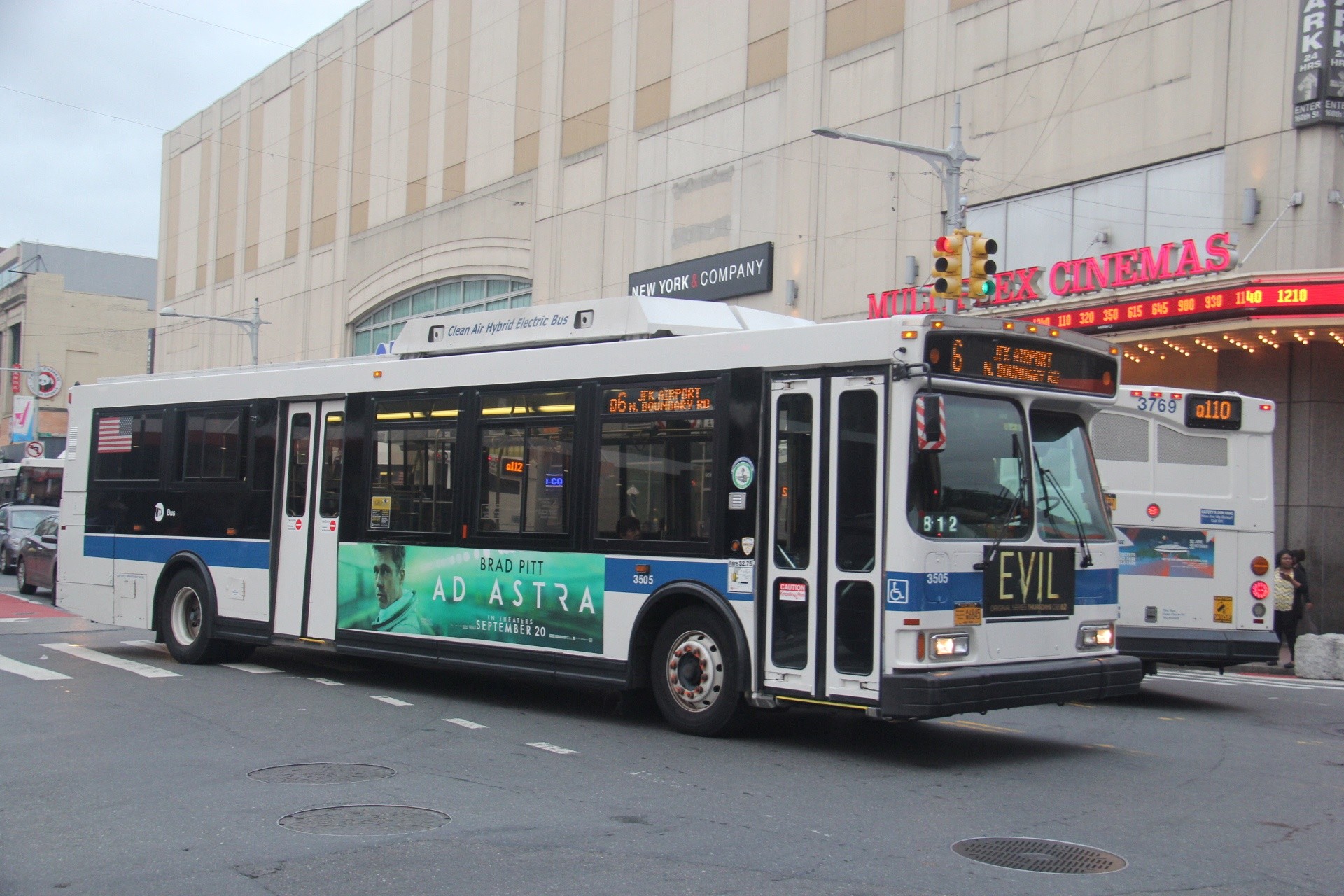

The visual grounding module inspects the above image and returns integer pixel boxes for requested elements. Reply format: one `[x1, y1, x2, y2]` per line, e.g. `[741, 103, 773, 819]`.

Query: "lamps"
[786, 280, 797, 306]
[906, 256, 919, 284]
[1241, 189, 1260, 223]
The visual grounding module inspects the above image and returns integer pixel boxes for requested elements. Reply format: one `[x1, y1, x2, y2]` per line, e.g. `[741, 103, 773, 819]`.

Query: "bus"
[999, 384, 1281, 679]
[55, 297, 1144, 740]
[13, 457, 65, 506]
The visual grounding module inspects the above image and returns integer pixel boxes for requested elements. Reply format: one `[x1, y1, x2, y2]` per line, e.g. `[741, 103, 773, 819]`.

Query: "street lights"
[158, 298, 273, 490]
[810, 93, 981, 314]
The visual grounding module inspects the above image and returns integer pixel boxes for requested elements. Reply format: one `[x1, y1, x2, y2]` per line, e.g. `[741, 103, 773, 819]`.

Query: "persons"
[617, 517, 645, 541]
[925, 446, 1075, 523]
[1267, 550, 1312, 672]
[369, 547, 423, 635]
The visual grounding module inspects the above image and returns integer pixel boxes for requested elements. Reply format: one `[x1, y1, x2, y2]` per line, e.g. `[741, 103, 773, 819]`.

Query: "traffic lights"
[969, 236, 997, 300]
[931, 246, 953, 300]
[934, 234, 964, 297]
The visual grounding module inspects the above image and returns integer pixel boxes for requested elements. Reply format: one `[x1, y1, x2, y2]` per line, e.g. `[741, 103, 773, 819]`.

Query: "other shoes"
[1267, 660, 1277, 665]
[1284, 662, 1294, 668]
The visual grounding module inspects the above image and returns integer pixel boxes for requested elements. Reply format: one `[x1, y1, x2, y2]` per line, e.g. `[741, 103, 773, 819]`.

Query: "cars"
[15, 513, 60, 596]
[0, 505, 60, 575]
